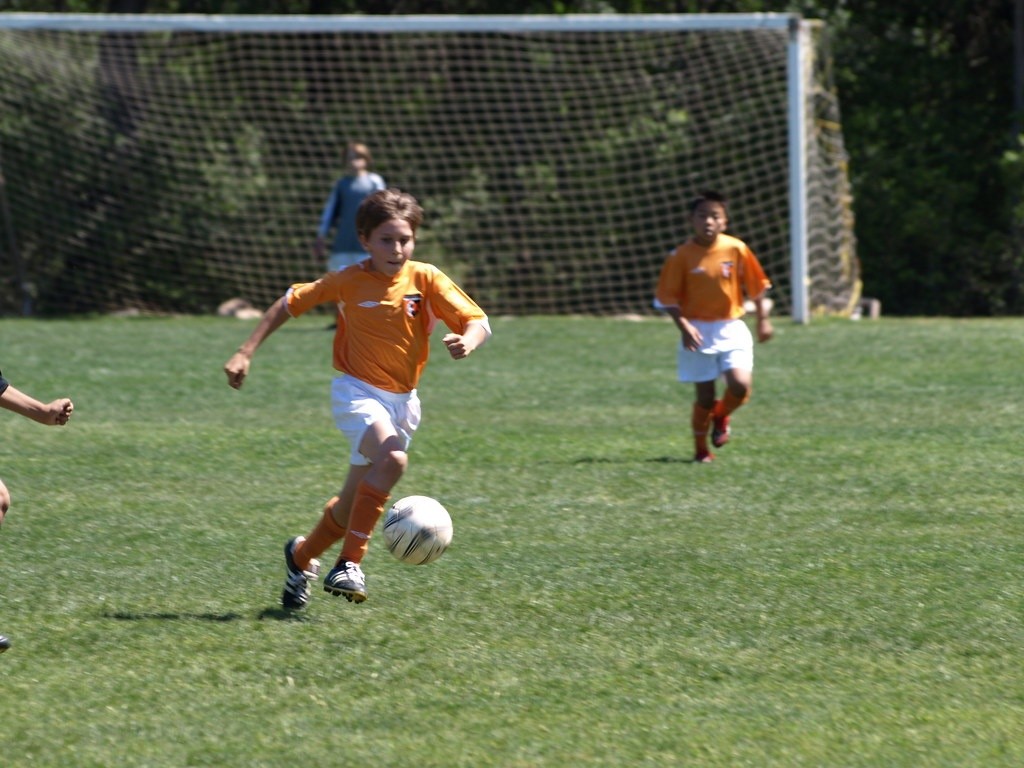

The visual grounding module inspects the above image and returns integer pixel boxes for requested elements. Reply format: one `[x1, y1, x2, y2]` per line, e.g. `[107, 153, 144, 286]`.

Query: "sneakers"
[323, 559, 369, 604]
[281, 535, 319, 610]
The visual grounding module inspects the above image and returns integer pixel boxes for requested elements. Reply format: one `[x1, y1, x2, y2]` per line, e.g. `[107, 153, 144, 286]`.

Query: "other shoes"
[711, 418, 728, 446]
[695, 453, 711, 463]
[0, 635, 11, 653]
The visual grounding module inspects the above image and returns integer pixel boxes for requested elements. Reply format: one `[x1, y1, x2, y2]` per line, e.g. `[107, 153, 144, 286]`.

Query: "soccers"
[379, 493, 457, 568]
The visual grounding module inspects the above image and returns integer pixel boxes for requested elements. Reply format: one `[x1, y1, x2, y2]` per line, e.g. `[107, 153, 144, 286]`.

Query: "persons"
[223, 143, 491, 609]
[0, 369, 74, 649]
[654, 191, 774, 463]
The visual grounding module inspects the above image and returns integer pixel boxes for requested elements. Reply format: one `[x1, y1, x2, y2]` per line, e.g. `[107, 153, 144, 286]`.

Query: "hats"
[348, 143, 371, 159]
